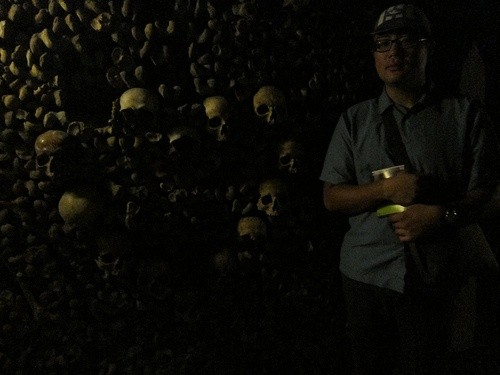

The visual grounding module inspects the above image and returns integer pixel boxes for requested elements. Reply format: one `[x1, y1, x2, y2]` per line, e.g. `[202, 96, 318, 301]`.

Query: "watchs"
[446, 206, 457, 227]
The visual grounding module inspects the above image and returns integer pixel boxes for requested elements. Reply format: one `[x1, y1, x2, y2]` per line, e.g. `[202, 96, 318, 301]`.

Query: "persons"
[318, 4, 500, 374]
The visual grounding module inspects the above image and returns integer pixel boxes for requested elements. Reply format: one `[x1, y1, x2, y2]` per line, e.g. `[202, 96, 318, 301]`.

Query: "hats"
[370, 3, 431, 35]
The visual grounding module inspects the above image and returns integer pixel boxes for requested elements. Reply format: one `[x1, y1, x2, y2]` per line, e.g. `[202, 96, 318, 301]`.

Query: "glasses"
[373, 36, 427, 53]
